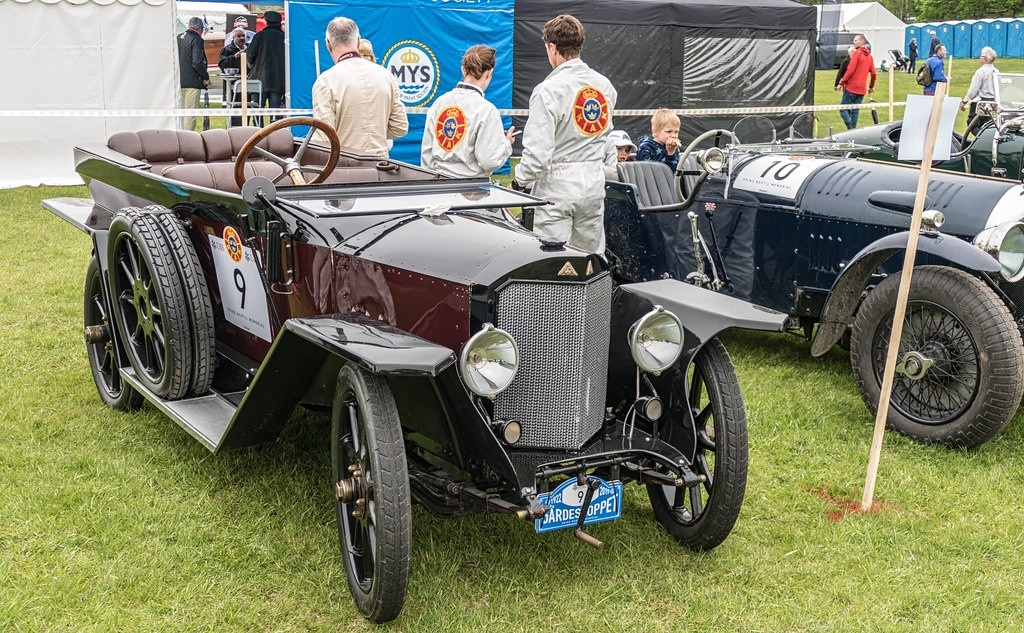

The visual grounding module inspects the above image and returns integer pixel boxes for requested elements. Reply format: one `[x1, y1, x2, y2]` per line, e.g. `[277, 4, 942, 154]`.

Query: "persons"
[636, 108, 681, 173]
[246, 11, 285, 128]
[420, 45, 515, 183]
[507, 15, 617, 263]
[176, 17, 210, 130]
[961, 46, 1000, 137]
[309, 17, 408, 158]
[879, 60, 887, 72]
[924, 44, 952, 96]
[834, 47, 856, 92]
[358, 39, 393, 152]
[234, 16, 249, 30]
[608, 130, 638, 162]
[837, 35, 877, 130]
[929, 31, 940, 58]
[907, 38, 918, 74]
[218, 27, 252, 127]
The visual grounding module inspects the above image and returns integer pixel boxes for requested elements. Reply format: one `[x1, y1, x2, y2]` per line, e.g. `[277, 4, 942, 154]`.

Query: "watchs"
[512, 180, 519, 190]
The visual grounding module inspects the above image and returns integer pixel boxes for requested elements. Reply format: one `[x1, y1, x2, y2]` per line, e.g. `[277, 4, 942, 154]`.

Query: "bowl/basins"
[224, 68, 239, 75]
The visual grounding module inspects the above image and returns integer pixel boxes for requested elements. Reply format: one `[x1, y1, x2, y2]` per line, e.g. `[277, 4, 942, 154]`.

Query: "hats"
[264, 11, 281, 23]
[189, 17, 205, 30]
[607, 130, 637, 153]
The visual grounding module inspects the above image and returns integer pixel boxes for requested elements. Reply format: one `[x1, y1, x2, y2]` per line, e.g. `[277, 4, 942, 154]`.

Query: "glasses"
[980, 56, 985, 59]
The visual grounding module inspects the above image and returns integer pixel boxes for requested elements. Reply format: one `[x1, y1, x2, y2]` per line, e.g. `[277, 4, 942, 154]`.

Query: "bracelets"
[962, 100, 967, 104]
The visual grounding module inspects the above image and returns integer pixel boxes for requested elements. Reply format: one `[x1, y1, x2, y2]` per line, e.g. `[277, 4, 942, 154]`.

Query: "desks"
[212, 75, 248, 120]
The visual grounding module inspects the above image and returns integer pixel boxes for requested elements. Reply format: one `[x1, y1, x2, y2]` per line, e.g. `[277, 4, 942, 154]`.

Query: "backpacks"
[916, 63, 933, 86]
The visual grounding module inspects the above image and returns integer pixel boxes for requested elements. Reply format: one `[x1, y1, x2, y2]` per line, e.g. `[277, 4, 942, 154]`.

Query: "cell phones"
[511, 130, 523, 137]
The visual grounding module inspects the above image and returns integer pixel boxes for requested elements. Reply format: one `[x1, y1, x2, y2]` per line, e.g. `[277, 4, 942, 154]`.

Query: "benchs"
[106, 121, 299, 193]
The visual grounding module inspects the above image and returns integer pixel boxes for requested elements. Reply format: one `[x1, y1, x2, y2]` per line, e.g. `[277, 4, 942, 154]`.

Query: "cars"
[41, 122, 789, 606]
[557, 118, 1021, 451]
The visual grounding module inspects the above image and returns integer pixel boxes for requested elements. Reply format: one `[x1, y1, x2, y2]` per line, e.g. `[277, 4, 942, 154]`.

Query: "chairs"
[228, 79, 263, 128]
[617, 161, 686, 206]
[682, 152, 706, 197]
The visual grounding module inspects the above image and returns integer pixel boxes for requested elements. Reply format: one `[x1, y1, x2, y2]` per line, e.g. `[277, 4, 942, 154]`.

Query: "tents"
[0, 0, 818, 190]
[813, 2, 1024, 69]
